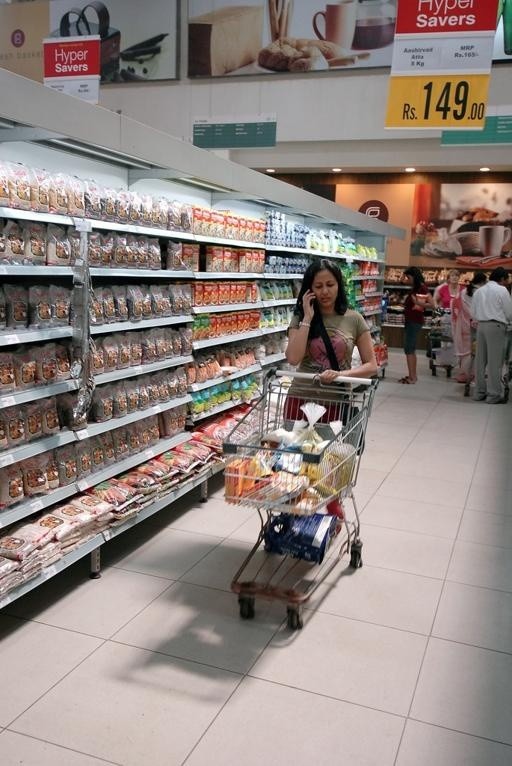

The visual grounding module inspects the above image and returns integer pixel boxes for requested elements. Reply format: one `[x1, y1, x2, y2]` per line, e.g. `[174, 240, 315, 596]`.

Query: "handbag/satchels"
[342, 406, 366, 457]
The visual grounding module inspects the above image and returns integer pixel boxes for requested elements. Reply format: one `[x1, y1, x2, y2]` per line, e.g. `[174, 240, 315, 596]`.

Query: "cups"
[480, 225, 511, 258]
[313, 4, 356, 52]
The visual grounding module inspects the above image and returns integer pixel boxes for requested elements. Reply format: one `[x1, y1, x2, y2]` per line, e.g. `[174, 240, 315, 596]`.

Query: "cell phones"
[308, 290, 313, 307]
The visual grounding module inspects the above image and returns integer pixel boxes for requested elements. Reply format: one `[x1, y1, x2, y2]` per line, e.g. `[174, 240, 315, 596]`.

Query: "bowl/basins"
[350, 17, 396, 51]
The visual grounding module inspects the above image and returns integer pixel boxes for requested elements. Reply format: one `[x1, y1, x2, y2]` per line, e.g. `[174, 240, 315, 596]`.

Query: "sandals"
[398, 375, 418, 384]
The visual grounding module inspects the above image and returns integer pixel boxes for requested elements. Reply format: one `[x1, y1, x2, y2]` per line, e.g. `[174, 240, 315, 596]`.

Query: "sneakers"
[473, 391, 507, 403]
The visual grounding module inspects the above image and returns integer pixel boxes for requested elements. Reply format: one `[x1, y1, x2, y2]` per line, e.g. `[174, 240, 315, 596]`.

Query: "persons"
[398, 266, 434, 385]
[453, 271, 486, 383]
[470, 266, 512, 403]
[431, 269, 464, 309]
[282, 258, 379, 536]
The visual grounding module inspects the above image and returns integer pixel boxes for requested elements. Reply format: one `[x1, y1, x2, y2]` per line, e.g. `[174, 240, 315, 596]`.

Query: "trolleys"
[464, 325, 512, 402]
[420, 307, 456, 378]
[220, 367, 379, 630]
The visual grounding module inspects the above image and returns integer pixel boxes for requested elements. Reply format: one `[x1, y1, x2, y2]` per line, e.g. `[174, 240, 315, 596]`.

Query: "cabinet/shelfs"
[267, 174, 405, 391]
[1, 66, 265, 610]
[380, 254, 512, 353]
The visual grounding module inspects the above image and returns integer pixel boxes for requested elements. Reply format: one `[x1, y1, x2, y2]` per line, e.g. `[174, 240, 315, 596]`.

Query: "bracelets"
[298, 321, 311, 327]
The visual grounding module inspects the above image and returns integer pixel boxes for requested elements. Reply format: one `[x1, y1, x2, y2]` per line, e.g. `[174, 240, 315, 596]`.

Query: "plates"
[255, 48, 384, 74]
